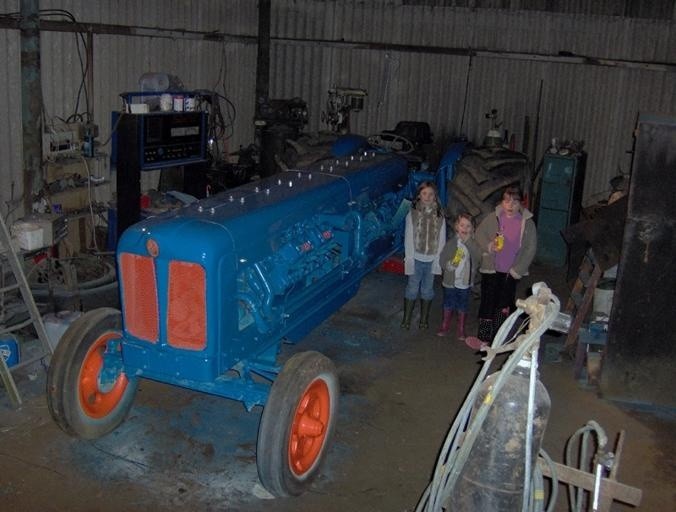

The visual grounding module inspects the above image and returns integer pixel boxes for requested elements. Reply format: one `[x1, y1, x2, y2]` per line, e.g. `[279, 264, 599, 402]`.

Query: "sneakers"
[463, 335, 489, 351]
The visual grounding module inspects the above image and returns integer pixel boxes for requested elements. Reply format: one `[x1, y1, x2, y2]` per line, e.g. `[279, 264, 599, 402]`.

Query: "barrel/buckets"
[0, 333, 22, 369]
[473, 109, 506, 146]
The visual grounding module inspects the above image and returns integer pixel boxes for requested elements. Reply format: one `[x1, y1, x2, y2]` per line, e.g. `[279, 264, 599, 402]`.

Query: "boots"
[436, 306, 453, 337]
[400, 297, 411, 327]
[455, 310, 467, 341]
[418, 298, 433, 331]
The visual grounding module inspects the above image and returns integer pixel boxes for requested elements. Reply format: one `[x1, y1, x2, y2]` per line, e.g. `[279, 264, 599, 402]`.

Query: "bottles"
[160, 94, 195, 113]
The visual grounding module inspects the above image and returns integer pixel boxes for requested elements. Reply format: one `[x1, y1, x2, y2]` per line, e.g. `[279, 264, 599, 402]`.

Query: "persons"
[474, 186, 537, 341]
[436, 212, 482, 341]
[400, 181, 446, 331]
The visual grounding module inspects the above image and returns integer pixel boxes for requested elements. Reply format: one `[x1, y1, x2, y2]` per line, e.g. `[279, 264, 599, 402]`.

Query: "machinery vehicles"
[45, 120, 530, 497]
